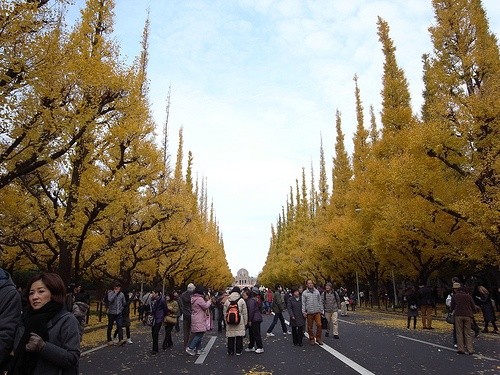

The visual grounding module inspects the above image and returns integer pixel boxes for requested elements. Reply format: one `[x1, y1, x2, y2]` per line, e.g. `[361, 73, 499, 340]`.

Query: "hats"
[452, 283, 460, 288]
[114, 282, 122, 286]
[188, 283, 195, 290]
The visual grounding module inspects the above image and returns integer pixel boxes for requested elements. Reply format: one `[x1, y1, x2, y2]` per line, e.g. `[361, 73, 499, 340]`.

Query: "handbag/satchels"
[143, 304, 150, 311]
[445, 313, 455, 324]
[164, 315, 177, 324]
[147, 314, 156, 326]
[320, 315, 328, 330]
[104, 292, 108, 311]
[490, 298, 499, 312]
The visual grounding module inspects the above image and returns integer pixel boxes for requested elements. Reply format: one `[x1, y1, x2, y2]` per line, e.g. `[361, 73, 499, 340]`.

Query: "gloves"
[26, 332, 46, 354]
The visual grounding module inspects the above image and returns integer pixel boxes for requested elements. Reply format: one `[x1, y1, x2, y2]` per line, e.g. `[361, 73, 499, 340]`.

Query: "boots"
[127, 338, 133, 344]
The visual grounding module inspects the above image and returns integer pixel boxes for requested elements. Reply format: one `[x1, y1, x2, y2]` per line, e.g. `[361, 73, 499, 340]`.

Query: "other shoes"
[186, 346, 196, 355]
[118, 340, 127, 346]
[255, 348, 264, 353]
[492, 328, 498, 333]
[196, 348, 206, 354]
[316, 340, 323, 345]
[310, 339, 315, 345]
[245, 347, 255, 351]
[107, 341, 114, 346]
[325, 332, 330, 337]
[236, 353, 241, 355]
[283, 329, 292, 335]
[481, 328, 488, 332]
[334, 335, 339, 339]
[267, 332, 275, 336]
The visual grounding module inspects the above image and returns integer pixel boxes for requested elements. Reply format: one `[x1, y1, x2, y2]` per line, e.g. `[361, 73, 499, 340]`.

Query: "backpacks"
[226, 296, 242, 325]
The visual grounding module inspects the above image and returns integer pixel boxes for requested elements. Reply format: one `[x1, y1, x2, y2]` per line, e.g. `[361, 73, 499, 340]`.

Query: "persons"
[3, 271, 81, 375]
[105, 279, 365, 355]
[66, 293, 89, 342]
[419, 284, 437, 329]
[0, 267, 22, 375]
[403, 285, 419, 329]
[445, 276, 500, 355]
[72, 285, 88, 304]
